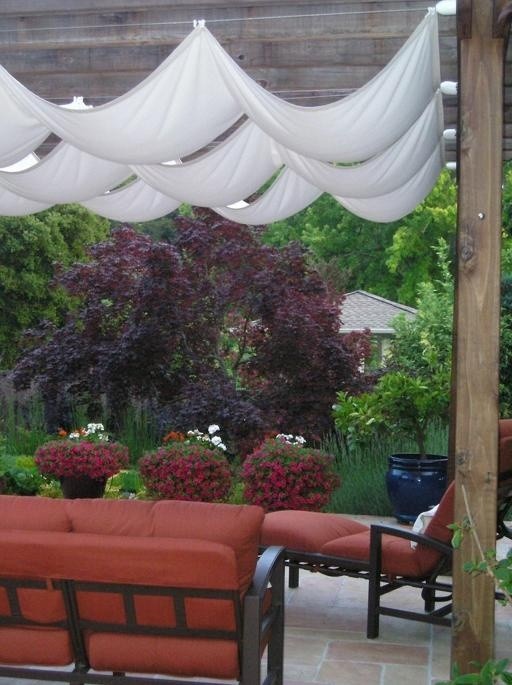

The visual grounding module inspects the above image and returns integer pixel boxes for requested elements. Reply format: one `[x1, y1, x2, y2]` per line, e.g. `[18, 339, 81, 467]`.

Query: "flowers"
[240, 434, 341, 513]
[33, 422, 130, 480]
[138, 422, 234, 505]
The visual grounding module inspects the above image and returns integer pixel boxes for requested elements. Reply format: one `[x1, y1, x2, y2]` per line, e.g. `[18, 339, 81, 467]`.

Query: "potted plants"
[326, 308, 454, 522]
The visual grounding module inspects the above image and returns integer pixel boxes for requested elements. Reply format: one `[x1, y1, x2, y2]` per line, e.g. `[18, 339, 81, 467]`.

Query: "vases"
[61, 476, 106, 499]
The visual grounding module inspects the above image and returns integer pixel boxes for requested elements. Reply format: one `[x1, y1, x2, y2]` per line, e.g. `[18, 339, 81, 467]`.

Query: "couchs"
[3, 494, 285, 685]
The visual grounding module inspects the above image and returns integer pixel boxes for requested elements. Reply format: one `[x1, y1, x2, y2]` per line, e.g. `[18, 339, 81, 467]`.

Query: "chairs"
[255, 418, 512, 638]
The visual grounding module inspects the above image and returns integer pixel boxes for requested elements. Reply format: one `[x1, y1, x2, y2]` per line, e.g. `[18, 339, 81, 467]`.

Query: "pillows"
[411, 504, 439, 550]
